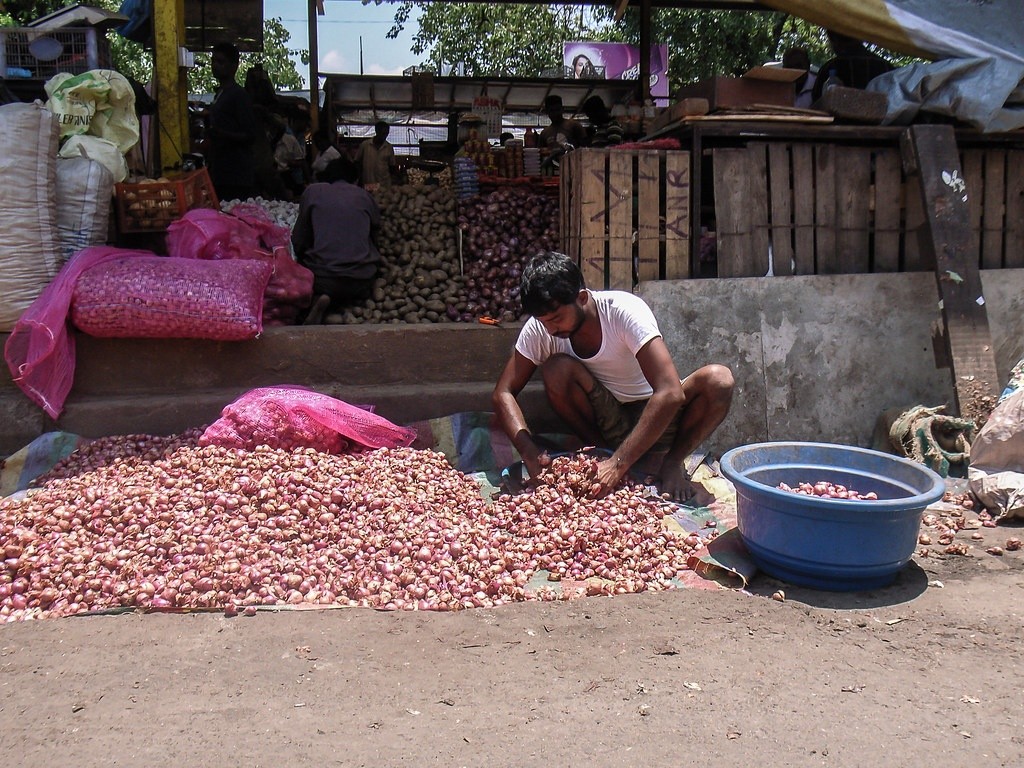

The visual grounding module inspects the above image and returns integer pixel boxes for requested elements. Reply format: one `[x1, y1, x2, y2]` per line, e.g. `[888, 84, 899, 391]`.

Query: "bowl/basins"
[720, 441, 945, 591]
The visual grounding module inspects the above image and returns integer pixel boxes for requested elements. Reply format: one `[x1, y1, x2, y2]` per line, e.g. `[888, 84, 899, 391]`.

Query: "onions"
[457, 188, 560, 322]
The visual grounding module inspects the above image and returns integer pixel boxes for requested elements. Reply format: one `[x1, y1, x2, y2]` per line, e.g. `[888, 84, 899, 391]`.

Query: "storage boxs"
[114, 168, 207, 232]
[689, 77, 798, 114]
[559, 147, 691, 292]
[0, 27, 112, 85]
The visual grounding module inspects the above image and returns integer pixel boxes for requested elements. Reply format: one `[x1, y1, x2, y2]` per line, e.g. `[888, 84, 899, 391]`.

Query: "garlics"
[67, 196, 315, 338]
[0, 384, 1024, 623]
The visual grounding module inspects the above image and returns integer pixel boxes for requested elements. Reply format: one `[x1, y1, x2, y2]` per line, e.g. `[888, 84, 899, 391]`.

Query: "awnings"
[317, 72, 644, 156]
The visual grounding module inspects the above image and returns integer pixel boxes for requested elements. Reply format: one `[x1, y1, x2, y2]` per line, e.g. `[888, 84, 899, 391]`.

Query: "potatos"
[407, 166, 453, 189]
[321, 182, 468, 324]
[125, 178, 207, 226]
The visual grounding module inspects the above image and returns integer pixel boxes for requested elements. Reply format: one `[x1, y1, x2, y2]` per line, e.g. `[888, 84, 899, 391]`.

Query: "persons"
[492, 252, 734, 504]
[208, 41, 401, 324]
[761, 29, 896, 108]
[500, 95, 624, 148]
